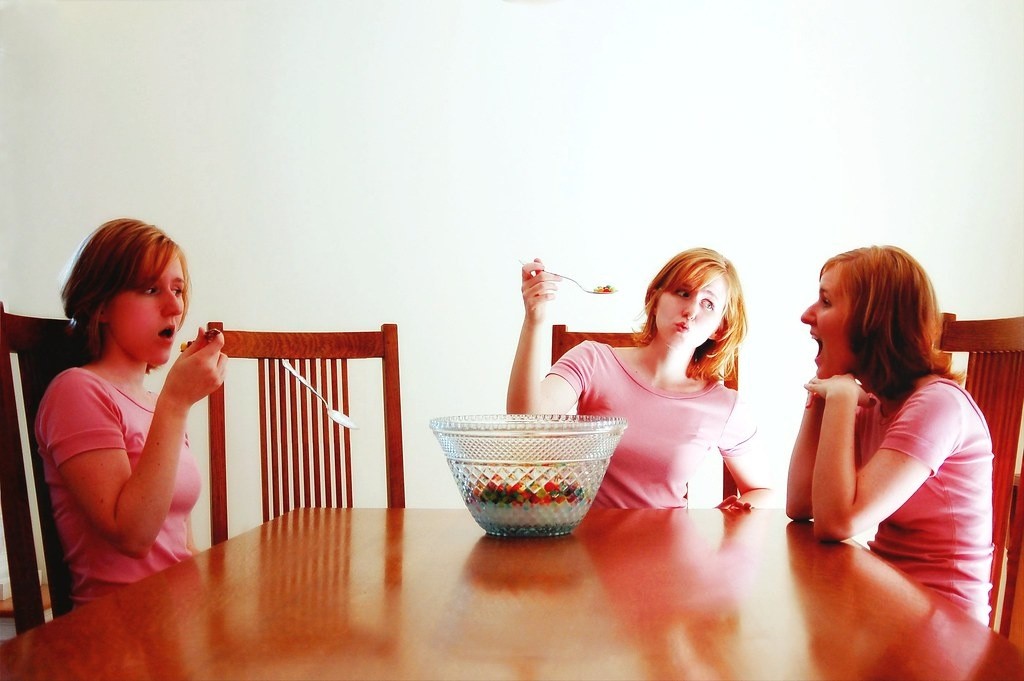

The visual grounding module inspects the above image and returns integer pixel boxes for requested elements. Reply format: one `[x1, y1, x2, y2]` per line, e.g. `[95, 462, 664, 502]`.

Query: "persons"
[785, 244, 995, 628]
[506, 247, 780, 509]
[33, 219, 229, 612]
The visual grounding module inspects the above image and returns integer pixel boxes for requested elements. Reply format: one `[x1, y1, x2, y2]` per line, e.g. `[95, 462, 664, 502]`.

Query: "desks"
[0, 503, 1024, 681]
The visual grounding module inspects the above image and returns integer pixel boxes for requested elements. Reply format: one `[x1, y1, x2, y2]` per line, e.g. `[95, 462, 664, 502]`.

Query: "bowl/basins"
[429, 415, 628, 539]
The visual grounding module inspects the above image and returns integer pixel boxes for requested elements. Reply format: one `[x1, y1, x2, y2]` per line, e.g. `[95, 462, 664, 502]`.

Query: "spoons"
[519, 260, 619, 295]
[281, 359, 356, 429]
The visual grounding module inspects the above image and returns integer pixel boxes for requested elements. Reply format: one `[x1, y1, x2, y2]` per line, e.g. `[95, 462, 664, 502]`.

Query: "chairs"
[0, 293, 84, 638]
[549, 322, 739, 509]
[206, 318, 408, 549]
[933, 311, 1024, 648]
